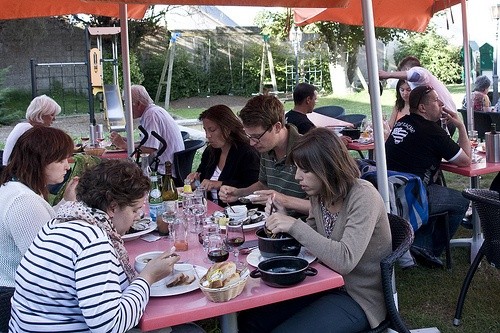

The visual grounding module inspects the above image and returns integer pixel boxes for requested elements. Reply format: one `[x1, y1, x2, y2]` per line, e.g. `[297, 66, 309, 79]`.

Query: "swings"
[227, 36, 246, 93]
[193, 35, 211, 98]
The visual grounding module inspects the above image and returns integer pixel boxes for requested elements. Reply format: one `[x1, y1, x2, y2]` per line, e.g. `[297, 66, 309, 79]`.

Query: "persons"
[284, 83, 353, 145]
[3, 94, 73, 195]
[378, 57, 457, 185]
[219, 94, 311, 223]
[241, 128, 392, 333]
[463, 74, 492, 111]
[383, 85, 472, 268]
[8, 160, 207, 333]
[186, 104, 257, 208]
[108, 84, 185, 189]
[0, 125, 81, 333]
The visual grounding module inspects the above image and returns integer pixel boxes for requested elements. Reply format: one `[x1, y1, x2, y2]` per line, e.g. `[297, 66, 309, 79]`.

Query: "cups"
[156, 187, 229, 262]
[362, 121, 370, 139]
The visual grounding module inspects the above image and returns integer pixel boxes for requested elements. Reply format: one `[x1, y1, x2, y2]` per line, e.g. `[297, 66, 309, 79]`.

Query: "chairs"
[362, 212, 414, 333]
[172, 131, 207, 186]
[453, 188, 500, 325]
[355, 157, 452, 270]
[458, 107, 500, 141]
[311, 105, 367, 159]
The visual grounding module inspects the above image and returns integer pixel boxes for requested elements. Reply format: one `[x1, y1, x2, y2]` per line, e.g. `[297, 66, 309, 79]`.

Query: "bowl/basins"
[256, 228, 302, 257]
[135, 251, 164, 273]
[228, 207, 248, 220]
[339, 129, 363, 140]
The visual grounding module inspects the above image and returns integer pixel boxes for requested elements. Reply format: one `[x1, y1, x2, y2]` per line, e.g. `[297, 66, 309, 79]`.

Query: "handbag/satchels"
[360, 160, 430, 231]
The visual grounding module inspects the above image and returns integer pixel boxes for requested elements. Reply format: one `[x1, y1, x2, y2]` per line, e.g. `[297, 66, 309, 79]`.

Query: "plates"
[133, 263, 208, 297]
[206, 210, 269, 230]
[246, 246, 316, 268]
[104, 149, 125, 152]
[121, 222, 157, 240]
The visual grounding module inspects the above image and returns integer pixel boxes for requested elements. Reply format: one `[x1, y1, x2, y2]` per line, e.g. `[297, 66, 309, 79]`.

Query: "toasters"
[161, 161, 179, 222]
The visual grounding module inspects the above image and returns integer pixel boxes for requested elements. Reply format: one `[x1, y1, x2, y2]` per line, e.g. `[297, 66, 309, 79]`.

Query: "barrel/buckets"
[90, 125, 104, 147]
[485, 132, 500, 162]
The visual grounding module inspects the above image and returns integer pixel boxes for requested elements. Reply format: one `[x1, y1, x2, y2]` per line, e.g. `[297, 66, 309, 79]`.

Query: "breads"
[166, 273, 196, 288]
[203, 261, 247, 286]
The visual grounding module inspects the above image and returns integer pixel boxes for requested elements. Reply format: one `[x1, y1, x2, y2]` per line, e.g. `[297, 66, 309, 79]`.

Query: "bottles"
[181, 179, 193, 214]
[491, 124, 496, 134]
[93, 119, 96, 126]
[148, 173, 163, 220]
[140, 155, 155, 203]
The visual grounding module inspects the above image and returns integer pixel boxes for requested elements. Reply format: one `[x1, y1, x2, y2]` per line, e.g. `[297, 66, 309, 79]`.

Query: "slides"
[102, 84, 126, 132]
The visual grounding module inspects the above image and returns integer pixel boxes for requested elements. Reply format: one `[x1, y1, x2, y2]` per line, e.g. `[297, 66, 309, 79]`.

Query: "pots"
[251, 255, 318, 288]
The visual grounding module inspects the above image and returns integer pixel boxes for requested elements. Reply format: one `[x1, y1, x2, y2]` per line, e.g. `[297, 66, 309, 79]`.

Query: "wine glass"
[81, 133, 90, 149]
[96, 132, 105, 147]
[471, 138, 480, 157]
[225, 220, 245, 268]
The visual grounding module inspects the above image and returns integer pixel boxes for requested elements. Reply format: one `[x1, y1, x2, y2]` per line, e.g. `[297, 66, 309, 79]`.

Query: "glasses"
[416, 86, 434, 107]
[242, 125, 270, 143]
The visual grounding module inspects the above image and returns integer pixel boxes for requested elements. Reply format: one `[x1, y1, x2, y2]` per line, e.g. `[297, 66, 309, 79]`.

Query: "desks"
[68, 142, 129, 164]
[439, 151, 500, 267]
[123, 188, 344, 333]
[285, 110, 354, 131]
